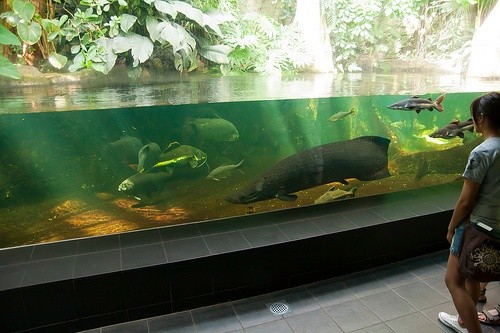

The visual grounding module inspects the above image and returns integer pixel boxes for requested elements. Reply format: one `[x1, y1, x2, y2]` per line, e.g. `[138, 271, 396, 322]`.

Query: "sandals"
[477, 304, 500, 326]
[478, 289, 487, 303]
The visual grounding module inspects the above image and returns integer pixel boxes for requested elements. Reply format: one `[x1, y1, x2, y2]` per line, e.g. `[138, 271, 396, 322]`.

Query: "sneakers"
[438, 312, 468, 333]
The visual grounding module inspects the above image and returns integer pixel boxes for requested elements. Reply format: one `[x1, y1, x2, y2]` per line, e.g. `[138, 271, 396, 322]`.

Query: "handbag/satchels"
[456, 221, 500, 282]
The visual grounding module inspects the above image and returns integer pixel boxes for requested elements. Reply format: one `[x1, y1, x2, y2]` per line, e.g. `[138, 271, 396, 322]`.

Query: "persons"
[437, 92, 500, 333]
[478, 304, 500, 323]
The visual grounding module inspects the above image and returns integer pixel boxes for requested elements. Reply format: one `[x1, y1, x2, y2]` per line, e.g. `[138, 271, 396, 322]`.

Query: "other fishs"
[4, 82, 478, 210]
